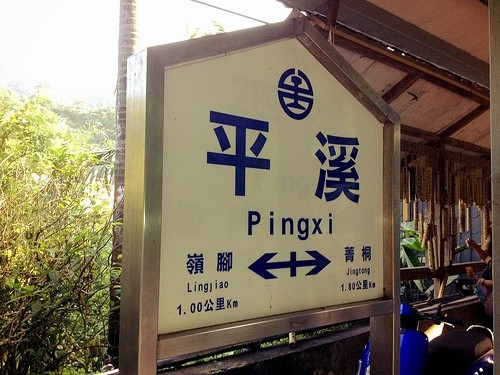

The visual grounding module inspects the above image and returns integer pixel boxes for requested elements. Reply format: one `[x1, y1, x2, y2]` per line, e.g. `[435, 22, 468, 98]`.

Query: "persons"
[464, 233, 493, 321]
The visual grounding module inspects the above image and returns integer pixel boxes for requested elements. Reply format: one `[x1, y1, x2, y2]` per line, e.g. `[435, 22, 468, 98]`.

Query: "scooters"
[356, 285, 494, 375]
